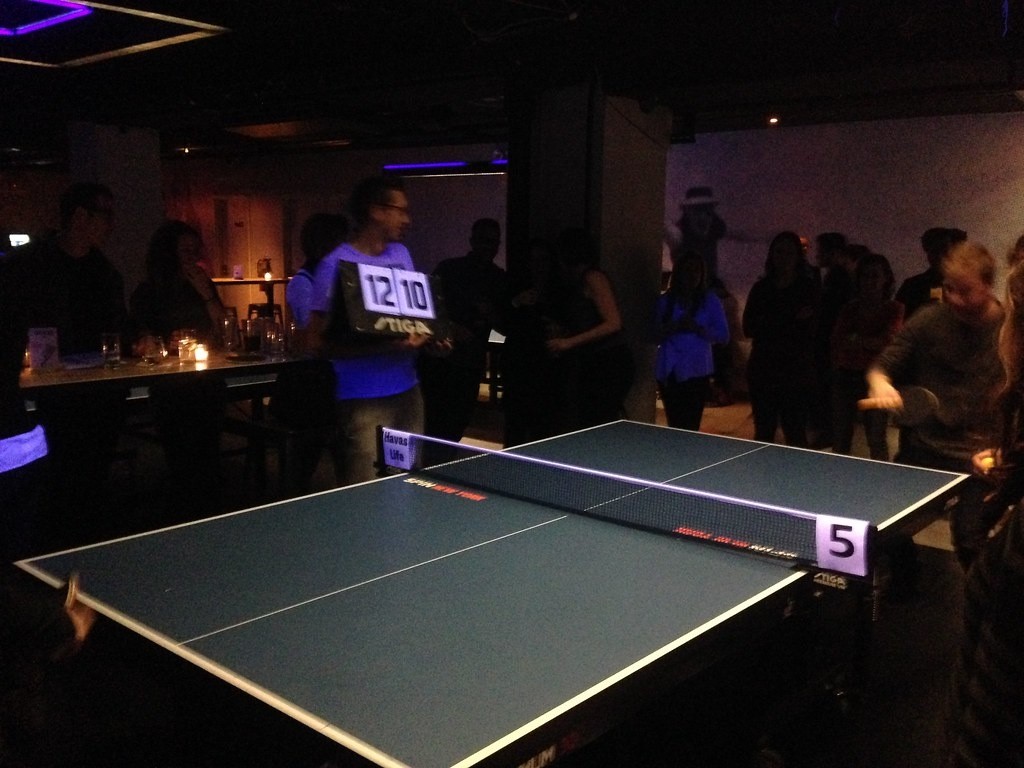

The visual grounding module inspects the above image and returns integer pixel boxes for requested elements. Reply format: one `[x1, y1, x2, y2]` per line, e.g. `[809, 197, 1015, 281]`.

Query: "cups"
[180, 329, 197, 363]
[101, 333, 120, 367]
[145, 336, 164, 366]
[224, 317, 298, 360]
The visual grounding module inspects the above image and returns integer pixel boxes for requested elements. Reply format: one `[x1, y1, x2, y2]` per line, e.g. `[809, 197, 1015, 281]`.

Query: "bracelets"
[205, 294, 222, 304]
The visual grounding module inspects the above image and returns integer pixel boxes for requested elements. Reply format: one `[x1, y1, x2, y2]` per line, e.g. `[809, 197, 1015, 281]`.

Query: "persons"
[525, 223, 635, 424]
[742, 232, 850, 451]
[131, 222, 241, 515]
[648, 247, 729, 432]
[867, 225, 1024, 768]
[306, 172, 432, 485]
[283, 211, 352, 495]
[430, 219, 511, 441]
[0, 183, 132, 530]
[831, 256, 905, 463]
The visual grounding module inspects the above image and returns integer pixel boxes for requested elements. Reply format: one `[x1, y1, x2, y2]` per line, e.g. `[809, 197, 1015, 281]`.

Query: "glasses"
[374, 202, 410, 215]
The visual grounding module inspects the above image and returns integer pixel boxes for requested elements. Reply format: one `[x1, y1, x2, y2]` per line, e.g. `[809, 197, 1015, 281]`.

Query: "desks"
[17, 345, 323, 537]
[13, 418, 973, 768]
[210, 277, 293, 339]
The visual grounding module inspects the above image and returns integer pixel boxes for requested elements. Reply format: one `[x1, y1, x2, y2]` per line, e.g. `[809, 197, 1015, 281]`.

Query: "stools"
[247, 303, 285, 340]
[221, 307, 240, 342]
[476, 341, 504, 405]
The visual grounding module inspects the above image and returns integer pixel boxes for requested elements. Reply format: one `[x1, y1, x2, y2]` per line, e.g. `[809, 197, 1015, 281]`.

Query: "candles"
[194, 346, 208, 362]
